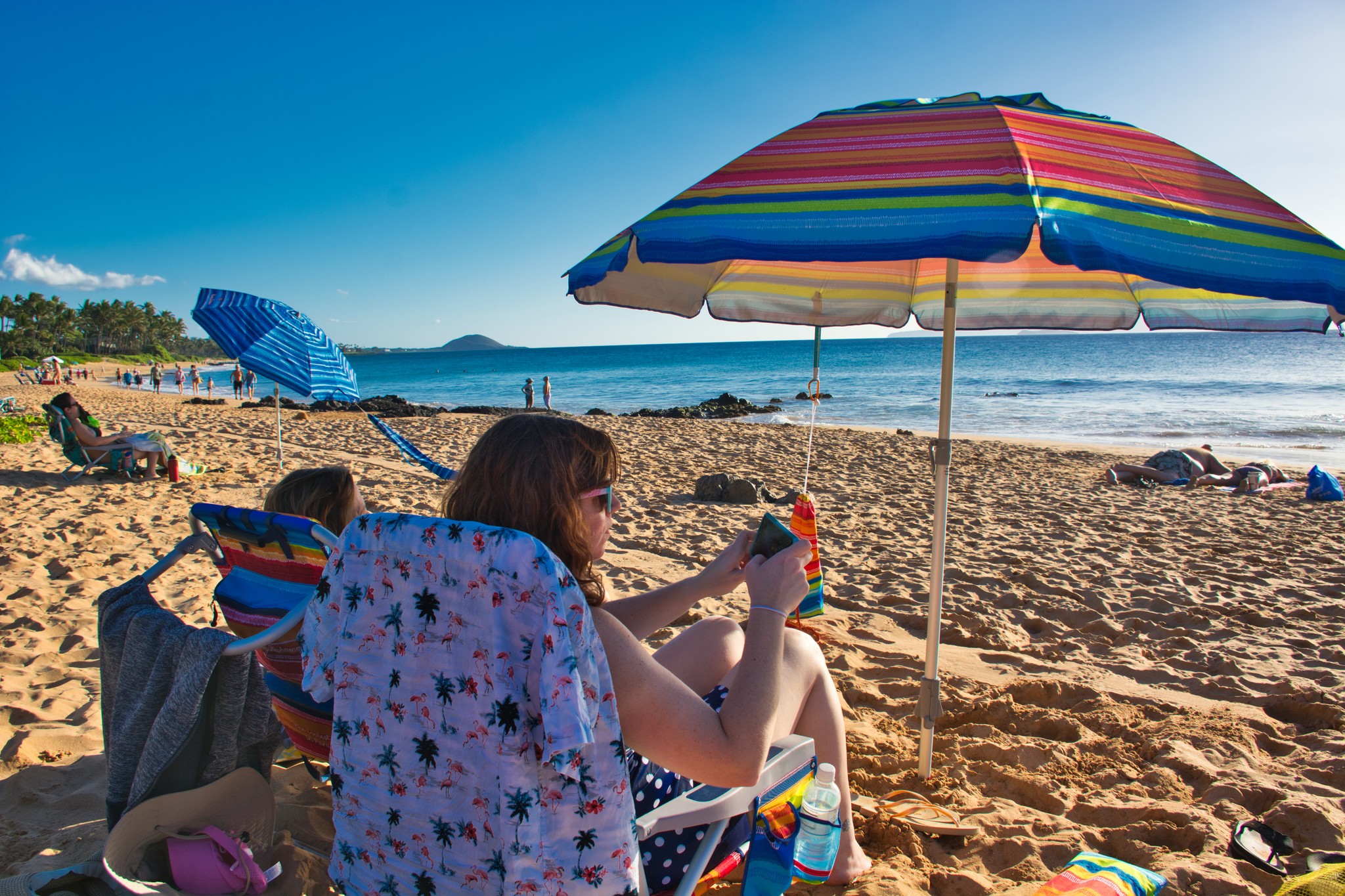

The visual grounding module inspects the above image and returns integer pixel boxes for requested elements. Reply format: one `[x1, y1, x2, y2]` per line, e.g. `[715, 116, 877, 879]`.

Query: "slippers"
[1228, 816, 1295, 876]
[851, 790, 981, 835]
[1307, 854, 1345, 879]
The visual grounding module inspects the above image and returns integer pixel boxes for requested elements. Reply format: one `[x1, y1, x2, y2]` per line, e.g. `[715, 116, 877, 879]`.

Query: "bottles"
[794, 763, 841, 880]
[125, 451, 132, 467]
[168, 454, 179, 482]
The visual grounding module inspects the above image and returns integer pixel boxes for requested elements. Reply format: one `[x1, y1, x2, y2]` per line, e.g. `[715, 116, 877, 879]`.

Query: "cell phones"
[750, 513, 799, 563]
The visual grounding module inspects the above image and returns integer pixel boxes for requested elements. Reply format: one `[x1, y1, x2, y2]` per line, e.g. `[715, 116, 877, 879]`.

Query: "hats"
[527, 378, 534, 382]
[137, 371, 140, 373]
[166, 828, 266, 896]
[103, 765, 277, 895]
[127, 369, 130, 371]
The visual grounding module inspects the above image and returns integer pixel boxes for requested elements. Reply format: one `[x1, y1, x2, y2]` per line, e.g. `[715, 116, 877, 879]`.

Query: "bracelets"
[750, 606, 787, 620]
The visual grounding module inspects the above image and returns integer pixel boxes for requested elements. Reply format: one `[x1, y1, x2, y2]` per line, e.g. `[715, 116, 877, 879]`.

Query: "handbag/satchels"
[1306, 464, 1343, 500]
[197, 376, 204, 384]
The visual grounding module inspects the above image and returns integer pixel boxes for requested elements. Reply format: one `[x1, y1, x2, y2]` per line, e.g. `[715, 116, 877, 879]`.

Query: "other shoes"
[206, 467, 226, 472]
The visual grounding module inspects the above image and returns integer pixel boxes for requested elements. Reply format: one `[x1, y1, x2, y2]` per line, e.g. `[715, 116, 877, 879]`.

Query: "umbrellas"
[71, 362, 79, 365]
[567, 91, 1345, 777]
[193, 288, 360, 470]
[43, 356, 64, 363]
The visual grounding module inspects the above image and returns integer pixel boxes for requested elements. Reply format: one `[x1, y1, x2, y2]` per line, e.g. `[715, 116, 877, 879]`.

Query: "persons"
[53, 358, 62, 385]
[207, 377, 215, 399]
[522, 378, 534, 408]
[261, 464, 370, 541]
[64, 367, 105, 387]
[543, 376, 551, 410]
[18, 361, 53, 382]
[174, 366, 186, 394]
[439, 414, 874, 896]
[230, 364, 244, 400]
[244, 370, 257, 400]
[1104, 444, 1290, 495]
[51, 393, 163, 478]
[187, 365, 200, 397]
[115, 362, 164, 394]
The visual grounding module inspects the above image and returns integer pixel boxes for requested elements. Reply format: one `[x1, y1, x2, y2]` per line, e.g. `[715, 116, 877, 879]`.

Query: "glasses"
[574, 476, 614, 515]
[70, 401, 80, 409]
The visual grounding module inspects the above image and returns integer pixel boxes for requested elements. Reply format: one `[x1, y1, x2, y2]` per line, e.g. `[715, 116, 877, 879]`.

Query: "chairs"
[40, 403, 207, 482]
[96, 503, 818, 896]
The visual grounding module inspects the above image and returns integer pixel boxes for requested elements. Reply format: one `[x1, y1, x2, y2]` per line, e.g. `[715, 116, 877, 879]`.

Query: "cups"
[1247, 471, 1260, 491]
